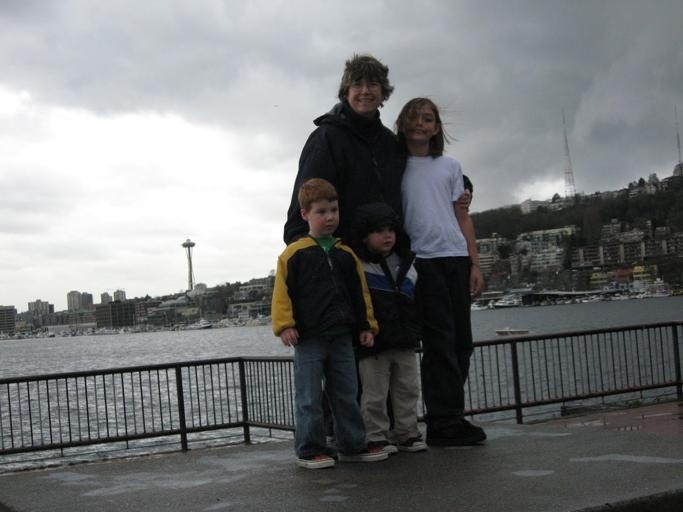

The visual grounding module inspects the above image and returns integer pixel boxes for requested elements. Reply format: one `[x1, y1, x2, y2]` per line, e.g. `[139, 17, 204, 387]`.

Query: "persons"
[269, 177, 389, 471]
[280, 55, 472, 244]
[396, 97, 488, 449]
[344, 199, 429, 455]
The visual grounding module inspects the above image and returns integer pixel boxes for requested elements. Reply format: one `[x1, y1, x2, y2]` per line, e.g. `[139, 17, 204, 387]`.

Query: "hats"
[354, 201, 400, 235]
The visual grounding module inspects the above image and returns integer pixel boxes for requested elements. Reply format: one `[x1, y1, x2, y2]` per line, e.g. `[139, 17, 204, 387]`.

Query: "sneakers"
[425, 418, 487, 447]
[292, 440, 427, 468]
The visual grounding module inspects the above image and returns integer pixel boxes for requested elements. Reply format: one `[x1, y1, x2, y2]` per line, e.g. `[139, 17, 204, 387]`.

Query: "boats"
[495, 327, 529, 336]
[493, 295, 519, 308]
[198, 320, 211, 328]
